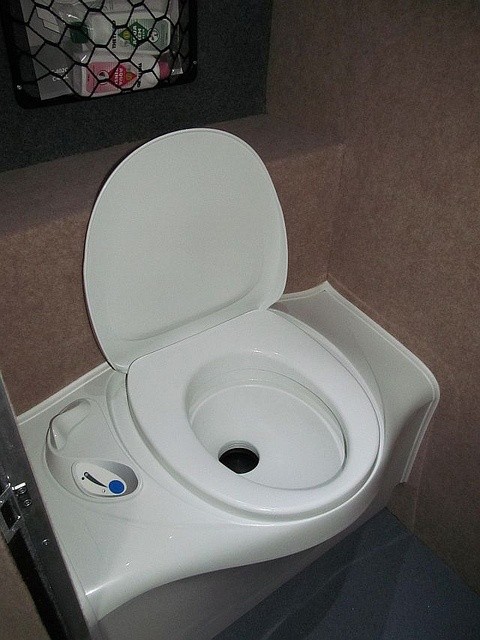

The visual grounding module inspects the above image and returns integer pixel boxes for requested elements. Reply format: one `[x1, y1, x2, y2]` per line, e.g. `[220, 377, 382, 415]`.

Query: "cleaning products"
[69, 10, 172, 51]
[73, 51, 171, 98]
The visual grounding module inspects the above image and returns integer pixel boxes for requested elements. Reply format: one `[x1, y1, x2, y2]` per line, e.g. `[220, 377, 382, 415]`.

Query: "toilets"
[15, 127, 442, 638]
